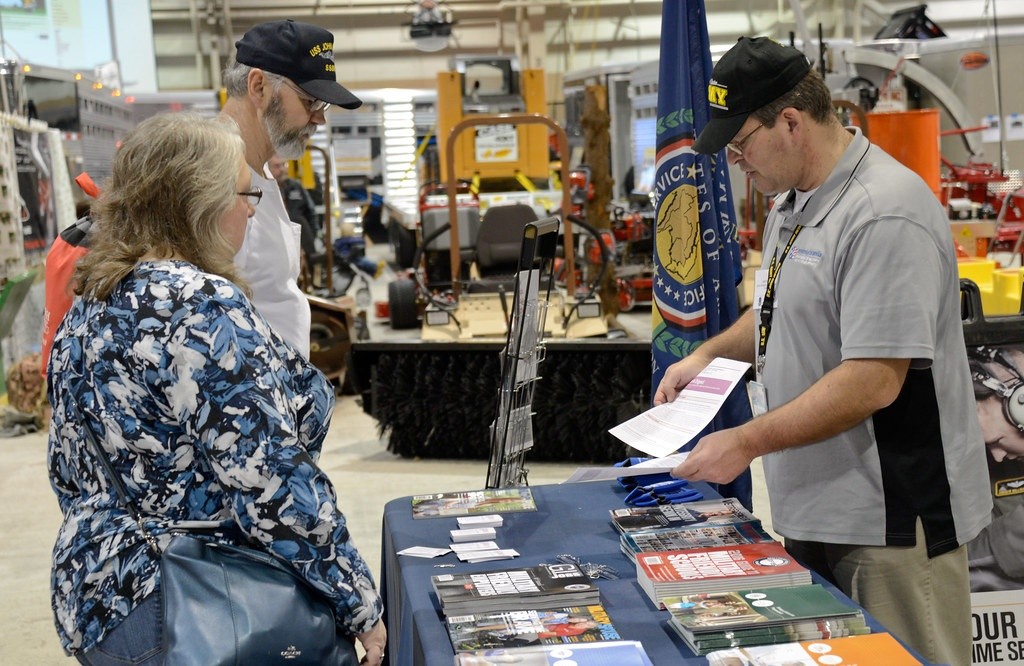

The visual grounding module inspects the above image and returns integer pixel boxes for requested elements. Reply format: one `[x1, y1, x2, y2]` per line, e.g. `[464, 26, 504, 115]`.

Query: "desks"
[378, 471, 934, 665]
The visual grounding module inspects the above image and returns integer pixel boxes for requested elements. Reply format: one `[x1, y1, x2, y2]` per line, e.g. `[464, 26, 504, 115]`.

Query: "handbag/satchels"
[148, 530, 338, 666]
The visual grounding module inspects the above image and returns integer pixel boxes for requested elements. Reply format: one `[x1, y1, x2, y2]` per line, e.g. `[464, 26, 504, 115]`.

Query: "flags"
[653, 0, 753, 511]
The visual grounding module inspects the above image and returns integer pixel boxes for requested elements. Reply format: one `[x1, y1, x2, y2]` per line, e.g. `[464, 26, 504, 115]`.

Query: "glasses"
[726, 114, 780, 154]
[279, 77, 332, 113]
[237, 186, 264, 210]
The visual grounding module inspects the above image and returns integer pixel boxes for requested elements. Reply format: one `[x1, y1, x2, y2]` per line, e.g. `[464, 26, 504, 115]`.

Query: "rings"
[380, 655, 384, 658]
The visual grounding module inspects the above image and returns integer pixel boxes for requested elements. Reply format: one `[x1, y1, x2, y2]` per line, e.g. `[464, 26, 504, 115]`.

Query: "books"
[446, 605, 624, 654]
[411, 488, 537, 519]
[432, 564, 602, 615]
[610, 497, 923, 666]
[453, 640, 654, 666]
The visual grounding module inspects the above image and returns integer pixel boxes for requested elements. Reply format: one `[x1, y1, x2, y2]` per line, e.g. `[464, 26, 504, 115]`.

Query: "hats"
[690, 36, 813, 155]
[233, 21, 363, 110]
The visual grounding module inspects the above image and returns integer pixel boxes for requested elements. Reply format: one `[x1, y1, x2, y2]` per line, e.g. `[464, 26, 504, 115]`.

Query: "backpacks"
[41, 171, 103, 380]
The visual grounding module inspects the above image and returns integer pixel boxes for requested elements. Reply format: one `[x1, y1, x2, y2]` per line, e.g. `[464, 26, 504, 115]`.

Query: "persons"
[45, 112, 387, 666]
[463, 612, 599, 644]
[442, 496, 525, 508]
[654, 36, 995, 666]
[968, 344, 1024, 585]
[213, 20, 359, 357]
[702, 600, 744, 607]
